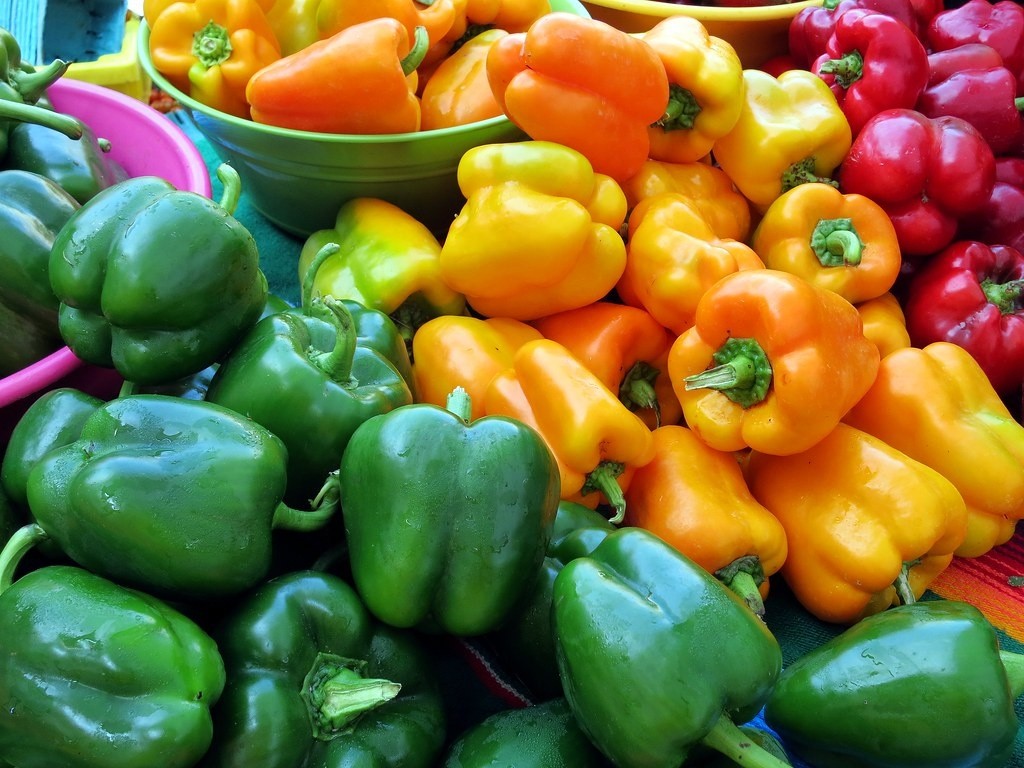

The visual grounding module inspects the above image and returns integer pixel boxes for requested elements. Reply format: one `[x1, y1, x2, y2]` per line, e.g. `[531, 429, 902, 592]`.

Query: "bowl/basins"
[0, 75, 213, 404]
[141, 0, 592, 234]
[582, 0, 836, 72]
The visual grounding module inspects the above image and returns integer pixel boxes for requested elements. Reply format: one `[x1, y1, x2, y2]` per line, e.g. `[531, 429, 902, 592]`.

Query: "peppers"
[0, 0, 1024, 768]
